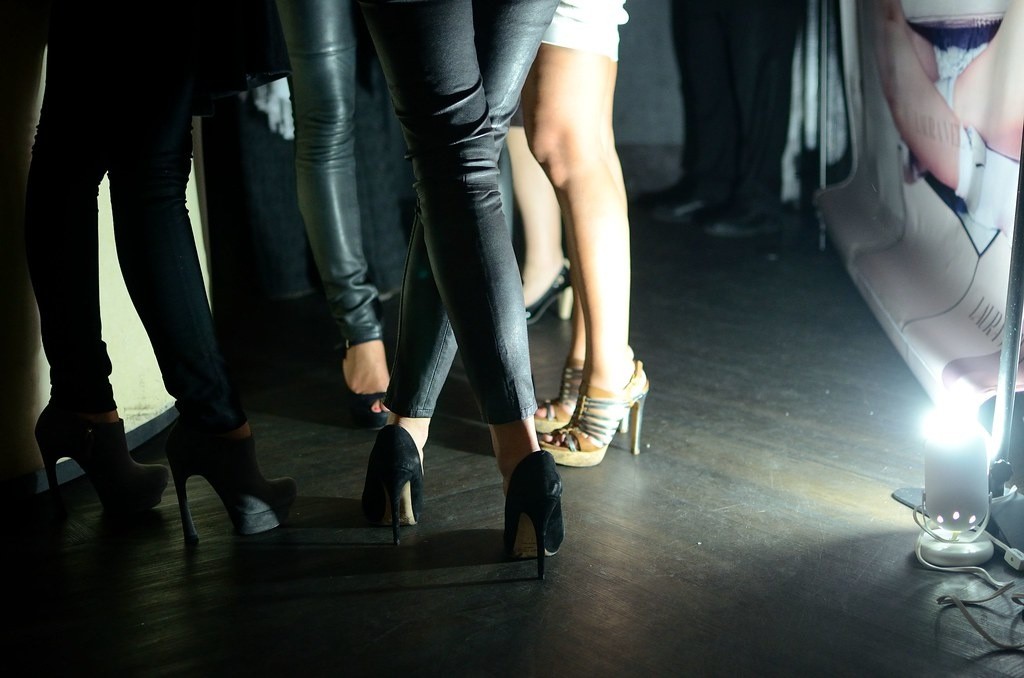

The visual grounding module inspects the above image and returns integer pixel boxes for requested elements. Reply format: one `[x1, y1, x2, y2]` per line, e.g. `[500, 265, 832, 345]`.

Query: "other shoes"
[702, 212, 785, 242]
[640, 189, 728, 223]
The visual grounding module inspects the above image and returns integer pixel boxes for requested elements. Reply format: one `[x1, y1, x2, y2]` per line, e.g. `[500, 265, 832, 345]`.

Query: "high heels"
[534, 366, 631, 434]
[333, 369, 390, 428]
[362, 423, 424, 548]
[503, 450, 565, 581]
[526, 266, 574, 325]
[538, 379, 649, 468]
[165, 416, 299, 549]
[34, 402, 168, 511]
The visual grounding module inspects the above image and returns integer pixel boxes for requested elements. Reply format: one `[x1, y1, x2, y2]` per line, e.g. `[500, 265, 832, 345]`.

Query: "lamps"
[913, 439, 995, 567]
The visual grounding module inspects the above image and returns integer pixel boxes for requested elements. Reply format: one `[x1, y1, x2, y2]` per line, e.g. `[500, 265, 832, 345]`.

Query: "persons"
[642, 0, 802, 237]
[864, 0, 1024, 243]
[0, 0, 648, 578]
